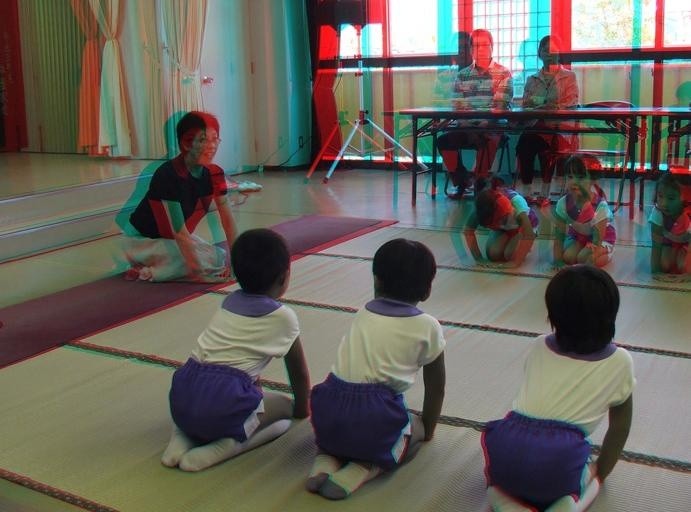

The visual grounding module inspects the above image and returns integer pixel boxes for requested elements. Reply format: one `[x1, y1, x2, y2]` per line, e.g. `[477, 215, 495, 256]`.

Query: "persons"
[436, 28, 514, 199]
[551, 153, 617, 272]
[160, 228, 310, 472]
[647, 165, 691, 283]
[516, 35, 579, 206]
[305, 238, 447, 500]
[481, 264, 638, 512]
[464, 176, 541, 269]
[121, 110, 239, 283]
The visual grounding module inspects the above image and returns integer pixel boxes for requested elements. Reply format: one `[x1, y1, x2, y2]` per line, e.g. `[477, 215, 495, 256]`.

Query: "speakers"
[305, 0, 341, 26]
[334, 0, 366, 25]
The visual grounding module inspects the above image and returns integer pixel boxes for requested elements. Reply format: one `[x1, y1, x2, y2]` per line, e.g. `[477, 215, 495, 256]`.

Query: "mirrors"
[0, 0, 179, 235]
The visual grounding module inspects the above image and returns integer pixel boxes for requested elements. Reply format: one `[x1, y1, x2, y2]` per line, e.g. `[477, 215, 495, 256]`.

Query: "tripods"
[323, 26, 432, 184]
[303, 26, 409, 183]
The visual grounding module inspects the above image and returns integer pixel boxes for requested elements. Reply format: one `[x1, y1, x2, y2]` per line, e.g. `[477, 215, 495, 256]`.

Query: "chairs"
[667, 102, 690, 173]
[444, 97, 513, 199]
[511, 102, 582, 207]
[563, 99, 638, 214]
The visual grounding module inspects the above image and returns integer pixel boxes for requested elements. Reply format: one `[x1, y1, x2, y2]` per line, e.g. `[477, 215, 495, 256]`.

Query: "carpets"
[0, 215, 399, 371]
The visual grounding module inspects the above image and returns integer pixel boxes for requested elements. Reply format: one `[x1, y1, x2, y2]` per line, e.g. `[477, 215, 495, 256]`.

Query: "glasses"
[197, 136, 222, 145]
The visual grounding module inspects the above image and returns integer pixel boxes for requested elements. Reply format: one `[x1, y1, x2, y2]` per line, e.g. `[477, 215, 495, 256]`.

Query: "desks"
[398, 106, 691, 207]
